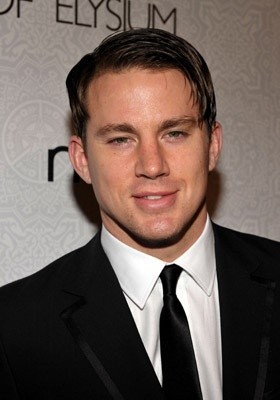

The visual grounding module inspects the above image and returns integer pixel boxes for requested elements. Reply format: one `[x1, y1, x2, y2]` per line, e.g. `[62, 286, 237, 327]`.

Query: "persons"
[0, 27, 279, 400]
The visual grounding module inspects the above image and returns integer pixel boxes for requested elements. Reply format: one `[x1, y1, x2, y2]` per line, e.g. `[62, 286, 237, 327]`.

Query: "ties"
[160, 264, 204, 400]
